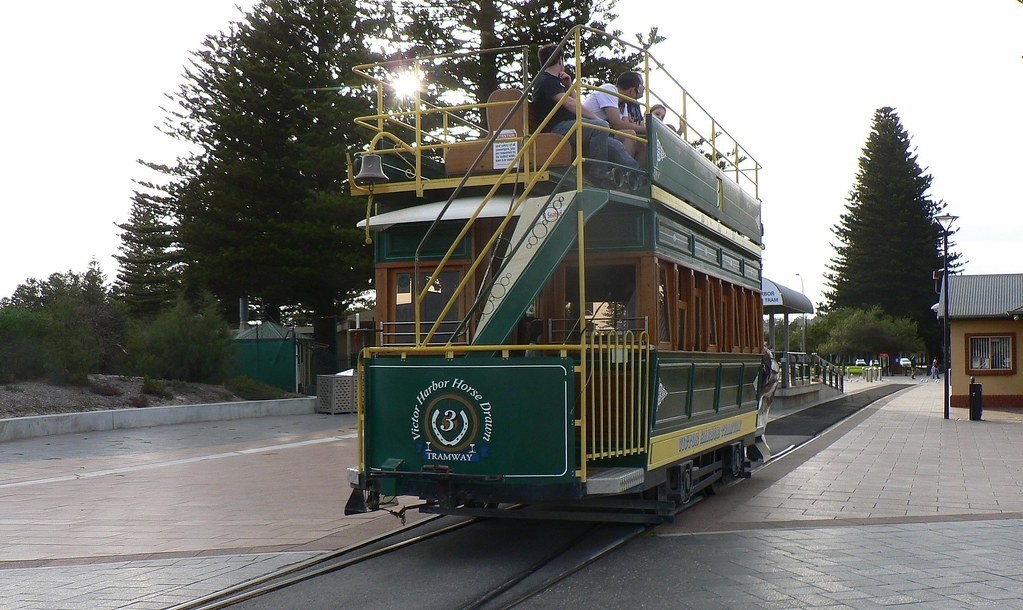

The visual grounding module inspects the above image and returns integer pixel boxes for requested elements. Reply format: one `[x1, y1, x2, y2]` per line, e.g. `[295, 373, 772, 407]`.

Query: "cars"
[899, 358, 912, 368]
[855, 358, 879, 366]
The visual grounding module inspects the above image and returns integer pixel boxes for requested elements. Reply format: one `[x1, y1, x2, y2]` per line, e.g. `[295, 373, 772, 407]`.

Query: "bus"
[342, 26, 772, 525]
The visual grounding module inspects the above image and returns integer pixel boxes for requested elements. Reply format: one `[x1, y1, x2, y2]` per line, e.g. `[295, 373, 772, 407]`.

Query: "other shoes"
[588, 173, 617, 190]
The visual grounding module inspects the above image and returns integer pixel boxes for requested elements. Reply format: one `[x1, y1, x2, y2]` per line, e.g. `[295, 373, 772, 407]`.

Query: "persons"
[630, 74, 648, 139]
[911, 355, 917, 379]
[931, 359, 941, 380]
[581, 70, 646, 168]
[531, 45, 647, 190]
[525, 319, 542, 357]
[650, 104, 686, 137]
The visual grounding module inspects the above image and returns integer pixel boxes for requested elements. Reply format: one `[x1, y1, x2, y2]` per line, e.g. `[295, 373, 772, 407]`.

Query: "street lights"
[934, 214, 959, 419]
[795, 272, 806, 364]
